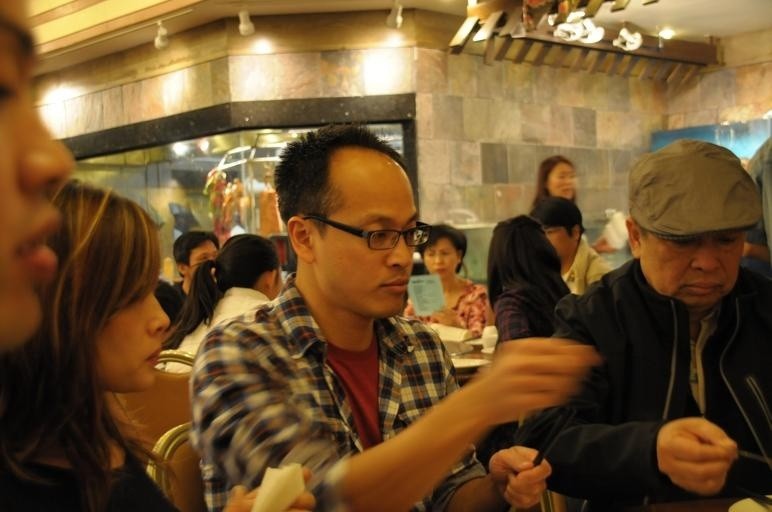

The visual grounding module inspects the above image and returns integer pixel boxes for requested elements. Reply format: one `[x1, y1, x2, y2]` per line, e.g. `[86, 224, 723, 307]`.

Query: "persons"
[538, 155, 616, 254]
[155, 234, 283, 375]
[1, 186, 178, 512]
[529, 196, 608, 297]
[519, 138, 771, 511]
[1, 2, 76, 348]
[405, 225, 492, 332]
[488, 216, 572, 344]
[743, 141, 770, 277]
[191, 123, 596, 512]
[155, 231, 220, 323]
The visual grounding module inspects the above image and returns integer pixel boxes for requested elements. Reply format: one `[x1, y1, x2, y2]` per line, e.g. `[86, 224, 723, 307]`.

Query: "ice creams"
[251, 463, 305, 512]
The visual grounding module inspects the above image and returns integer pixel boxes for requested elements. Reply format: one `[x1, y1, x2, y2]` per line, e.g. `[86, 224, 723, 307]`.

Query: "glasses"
[302, 221, 433, 251]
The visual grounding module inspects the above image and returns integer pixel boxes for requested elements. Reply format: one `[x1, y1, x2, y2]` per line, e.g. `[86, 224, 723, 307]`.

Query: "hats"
[627, 137, 761, 239]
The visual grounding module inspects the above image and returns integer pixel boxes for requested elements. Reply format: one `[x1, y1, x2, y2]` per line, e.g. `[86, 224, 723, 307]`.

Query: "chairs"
[109, 347, 205, 512]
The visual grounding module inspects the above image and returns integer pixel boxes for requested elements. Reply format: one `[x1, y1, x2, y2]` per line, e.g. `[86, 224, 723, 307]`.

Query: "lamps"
[385, 5, 403, 28]
[154, 20, 169, 49]
[237, 11, 256, 37]
